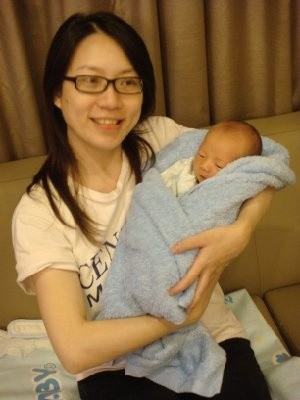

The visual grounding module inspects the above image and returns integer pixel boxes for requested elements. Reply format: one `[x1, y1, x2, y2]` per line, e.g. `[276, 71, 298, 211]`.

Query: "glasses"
[63, 75, 144, 94]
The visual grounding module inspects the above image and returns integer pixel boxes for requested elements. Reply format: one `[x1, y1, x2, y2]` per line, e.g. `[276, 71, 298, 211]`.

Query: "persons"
[12, 12, 274, 400]
[94, 121, 296, 398]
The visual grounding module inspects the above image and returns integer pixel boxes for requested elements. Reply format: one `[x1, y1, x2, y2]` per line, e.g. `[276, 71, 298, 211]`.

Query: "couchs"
[0, 112, 300, 357]
